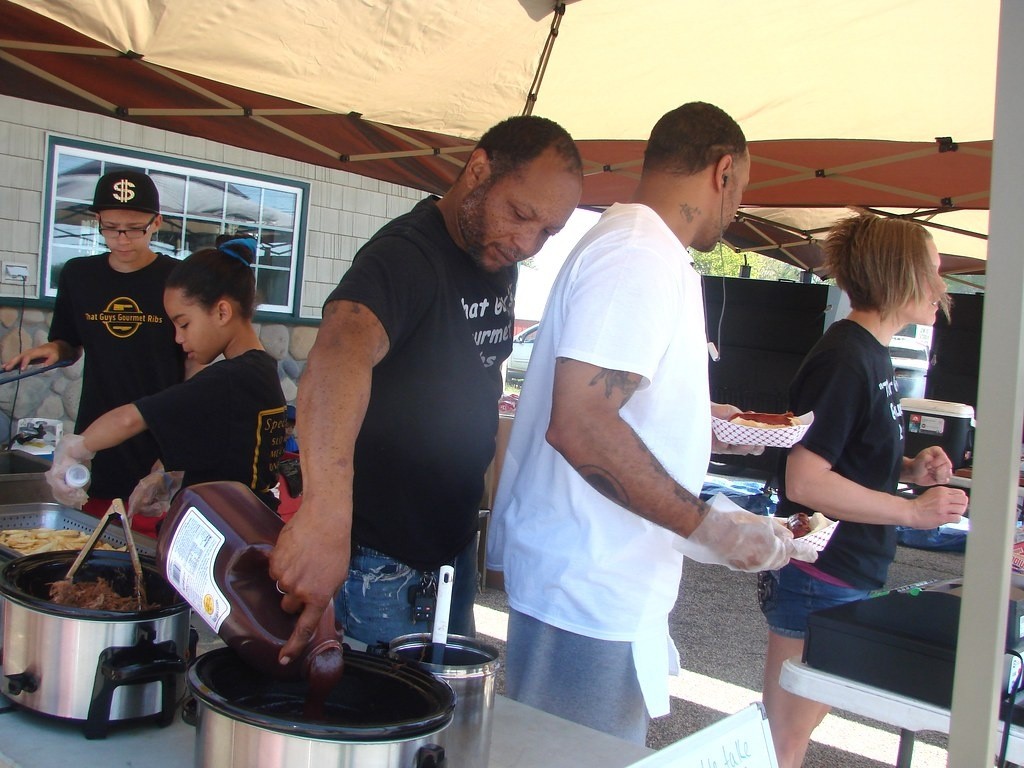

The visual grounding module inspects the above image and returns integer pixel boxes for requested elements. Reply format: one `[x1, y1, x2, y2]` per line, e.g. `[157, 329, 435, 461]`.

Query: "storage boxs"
[772, 513, 840, 552]
[805, 593, 1024, 708]
[900, 398, 976, 469]
[713, 409, 817, 447]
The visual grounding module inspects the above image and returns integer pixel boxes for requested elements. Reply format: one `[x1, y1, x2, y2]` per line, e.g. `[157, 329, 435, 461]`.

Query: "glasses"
[99, 215, 156, 239]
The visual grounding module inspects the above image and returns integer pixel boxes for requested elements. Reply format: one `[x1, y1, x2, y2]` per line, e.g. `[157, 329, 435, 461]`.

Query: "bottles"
[65, 464, 89, 488]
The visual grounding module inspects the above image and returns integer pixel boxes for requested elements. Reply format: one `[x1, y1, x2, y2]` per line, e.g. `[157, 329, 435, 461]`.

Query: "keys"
[407, 582, 437, 625]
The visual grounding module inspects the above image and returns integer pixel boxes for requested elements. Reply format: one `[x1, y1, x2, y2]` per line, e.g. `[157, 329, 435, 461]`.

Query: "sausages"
[786, 513, 811, 539]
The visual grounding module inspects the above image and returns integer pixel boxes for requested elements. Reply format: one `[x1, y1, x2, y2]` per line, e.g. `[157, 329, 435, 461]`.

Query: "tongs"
[63, 498, 148, 611]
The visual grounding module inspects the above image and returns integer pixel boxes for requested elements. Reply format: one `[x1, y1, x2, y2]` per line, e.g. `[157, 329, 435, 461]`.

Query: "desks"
[952, 467, 1024, 501]
[778, 653, 1024, 768]
[0, 652, 662, 768]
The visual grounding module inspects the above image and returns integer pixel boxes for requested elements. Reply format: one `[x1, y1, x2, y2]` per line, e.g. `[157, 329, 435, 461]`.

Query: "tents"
[0, 0, 1024, 767]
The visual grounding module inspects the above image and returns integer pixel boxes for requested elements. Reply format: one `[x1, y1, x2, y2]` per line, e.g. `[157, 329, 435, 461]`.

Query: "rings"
[276, 581, 286, 595]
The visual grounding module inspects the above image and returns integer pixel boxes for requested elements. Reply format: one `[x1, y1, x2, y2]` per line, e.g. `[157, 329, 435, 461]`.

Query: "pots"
[182, 644, 456, 768]
[0, 549, 198, 737]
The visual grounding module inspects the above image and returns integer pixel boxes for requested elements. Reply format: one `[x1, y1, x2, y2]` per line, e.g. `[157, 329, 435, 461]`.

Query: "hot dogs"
[727, 411, 802, 429]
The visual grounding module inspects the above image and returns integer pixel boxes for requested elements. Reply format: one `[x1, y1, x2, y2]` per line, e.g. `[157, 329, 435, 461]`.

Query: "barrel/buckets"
[156, 482, 345, 681]
[365, 633, 500, 766]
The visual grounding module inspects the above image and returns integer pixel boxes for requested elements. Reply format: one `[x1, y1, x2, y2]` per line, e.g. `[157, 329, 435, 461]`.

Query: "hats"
[88, 172, 159, 215]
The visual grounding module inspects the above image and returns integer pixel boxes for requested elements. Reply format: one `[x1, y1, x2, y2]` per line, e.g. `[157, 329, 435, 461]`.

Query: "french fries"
[0, 527, 128, 557]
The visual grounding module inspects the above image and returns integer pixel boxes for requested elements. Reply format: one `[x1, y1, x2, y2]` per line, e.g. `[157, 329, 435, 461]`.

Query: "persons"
[285, 405, 299, 453]
[269, 117, 584, 668]
[485, 102, 793, 747]
[759, 213, 969, 768]
[0, 170, 209, 539]
[45, 233, 286, 519]
